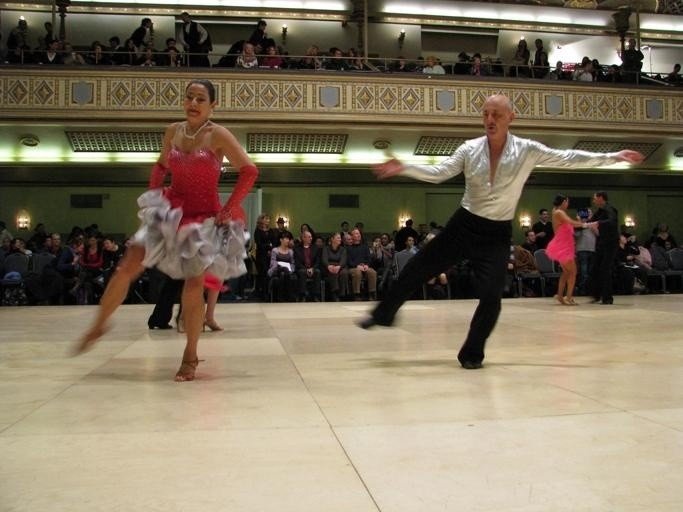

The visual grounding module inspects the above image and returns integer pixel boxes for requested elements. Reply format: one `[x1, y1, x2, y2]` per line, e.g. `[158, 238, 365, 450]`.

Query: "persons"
[235, 20, 447, 74]
[506, 192, 681, 305]
[0, 221, 158, 305]
[353, 96, 645, 369]
[148, 275, 226, 330]
[6, 10, 212, 69]
[220, 213, 477, 303]
[470, 39, 682, 87]
[60, 78, 260, 381]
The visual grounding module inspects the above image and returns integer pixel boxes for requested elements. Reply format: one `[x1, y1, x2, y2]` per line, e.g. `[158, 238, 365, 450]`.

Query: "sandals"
[66, 324, 106, 356]
[174, 354, 201, 381]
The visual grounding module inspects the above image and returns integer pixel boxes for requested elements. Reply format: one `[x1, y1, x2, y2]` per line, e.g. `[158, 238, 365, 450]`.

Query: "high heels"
[554, 294, 579, 306]
[202, 317, 224, 332]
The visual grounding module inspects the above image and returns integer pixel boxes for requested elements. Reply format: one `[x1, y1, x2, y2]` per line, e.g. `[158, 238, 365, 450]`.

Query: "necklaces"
[183, 119, 209, 139]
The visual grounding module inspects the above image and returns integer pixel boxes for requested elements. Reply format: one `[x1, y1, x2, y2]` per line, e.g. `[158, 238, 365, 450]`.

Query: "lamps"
[280, 24, 288, 44]
[397, 30, 405, 44]
[17, 15, 27, 28]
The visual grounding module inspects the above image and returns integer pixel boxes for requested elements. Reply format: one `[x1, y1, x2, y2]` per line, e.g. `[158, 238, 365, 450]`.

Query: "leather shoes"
[354, 312, 391, 331]
[148, 320, 174, 330]
[587, 297, 615, 305]
[457, 351, 484, 369]
[232, 290, 375, 303]
